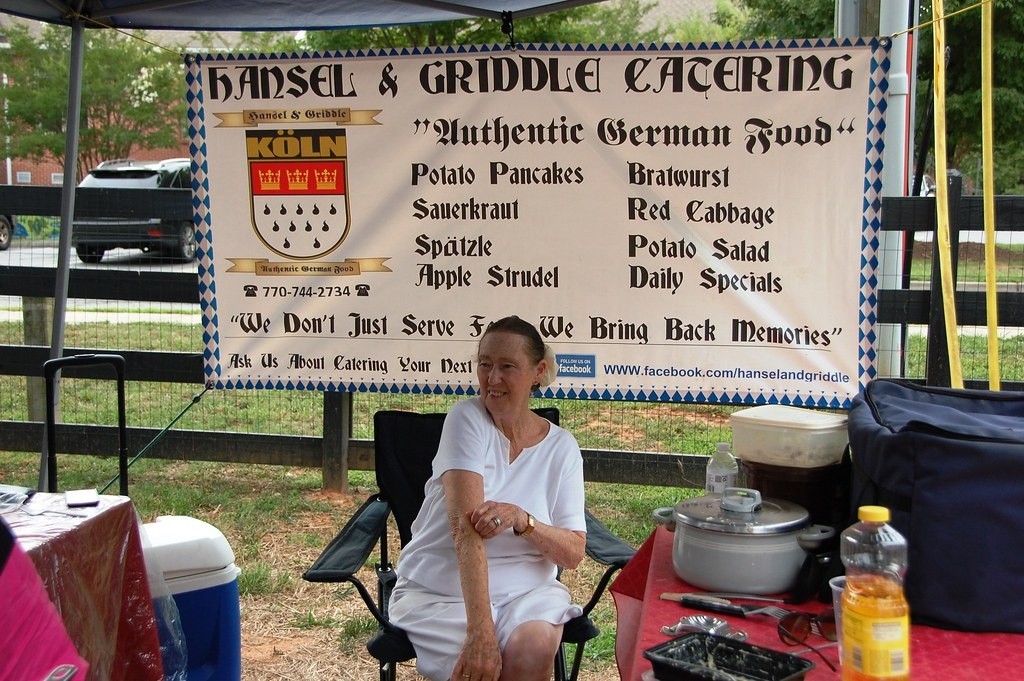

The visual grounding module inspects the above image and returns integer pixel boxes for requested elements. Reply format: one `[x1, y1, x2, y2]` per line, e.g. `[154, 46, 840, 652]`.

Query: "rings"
[491, 515, 505, 527]
[462, 673, 470, 678]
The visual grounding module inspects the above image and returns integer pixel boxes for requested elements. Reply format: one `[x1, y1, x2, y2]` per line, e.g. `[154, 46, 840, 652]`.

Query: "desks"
[608, 516, 1024, 681]
[0, 485, 192, 681]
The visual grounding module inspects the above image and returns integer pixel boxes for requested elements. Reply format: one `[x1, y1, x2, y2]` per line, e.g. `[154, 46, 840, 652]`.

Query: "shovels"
[680, 596, 821, 637]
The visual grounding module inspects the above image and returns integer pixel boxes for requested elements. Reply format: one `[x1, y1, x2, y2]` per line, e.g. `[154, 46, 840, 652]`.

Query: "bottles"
[840, 505, 911, 680]
[706, 442, 739, 493]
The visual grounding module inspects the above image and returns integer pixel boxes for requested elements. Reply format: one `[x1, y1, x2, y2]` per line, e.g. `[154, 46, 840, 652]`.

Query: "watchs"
[513, 511, 536, 538]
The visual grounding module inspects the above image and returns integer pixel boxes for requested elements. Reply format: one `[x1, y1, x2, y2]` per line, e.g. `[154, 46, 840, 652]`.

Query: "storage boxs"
[730, 403, 847, 469]
[137, 514, 244, 681]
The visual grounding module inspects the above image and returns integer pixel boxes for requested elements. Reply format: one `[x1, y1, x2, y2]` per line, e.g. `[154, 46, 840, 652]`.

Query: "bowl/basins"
[644, 632, 817, 681]
[731, 405, 849, 469]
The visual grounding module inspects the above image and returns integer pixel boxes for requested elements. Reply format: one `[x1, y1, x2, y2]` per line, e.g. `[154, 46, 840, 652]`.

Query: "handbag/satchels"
[839, 375, 1023, 634]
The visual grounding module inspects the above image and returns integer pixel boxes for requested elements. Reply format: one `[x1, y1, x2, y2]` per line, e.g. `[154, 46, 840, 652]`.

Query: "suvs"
[71, 157, 196, 264]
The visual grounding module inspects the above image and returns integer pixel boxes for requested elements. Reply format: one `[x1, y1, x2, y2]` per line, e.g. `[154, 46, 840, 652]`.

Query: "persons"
[388, 316, 587, 681]
[0, 517, 91, 681]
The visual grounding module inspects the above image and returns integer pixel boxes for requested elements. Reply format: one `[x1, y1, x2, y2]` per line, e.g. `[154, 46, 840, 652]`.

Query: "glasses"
[778, 607, 841, 672]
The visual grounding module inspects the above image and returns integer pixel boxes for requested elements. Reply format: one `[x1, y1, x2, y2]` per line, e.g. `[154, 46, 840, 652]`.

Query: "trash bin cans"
[137, 514, 242, 681]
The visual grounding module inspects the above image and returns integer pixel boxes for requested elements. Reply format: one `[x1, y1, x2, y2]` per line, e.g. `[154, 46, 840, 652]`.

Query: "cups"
[829, 575, 847, 666]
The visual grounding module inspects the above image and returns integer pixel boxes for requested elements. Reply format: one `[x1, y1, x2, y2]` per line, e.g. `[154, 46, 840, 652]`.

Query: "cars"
[0, 214, 17, 252]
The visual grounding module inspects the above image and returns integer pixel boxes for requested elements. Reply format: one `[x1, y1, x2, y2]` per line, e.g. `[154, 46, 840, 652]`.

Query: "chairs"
[302, 405, 637, 680]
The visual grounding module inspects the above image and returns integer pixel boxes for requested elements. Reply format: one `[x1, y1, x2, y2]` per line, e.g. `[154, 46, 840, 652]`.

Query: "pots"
[652, 487, 833, 596]
